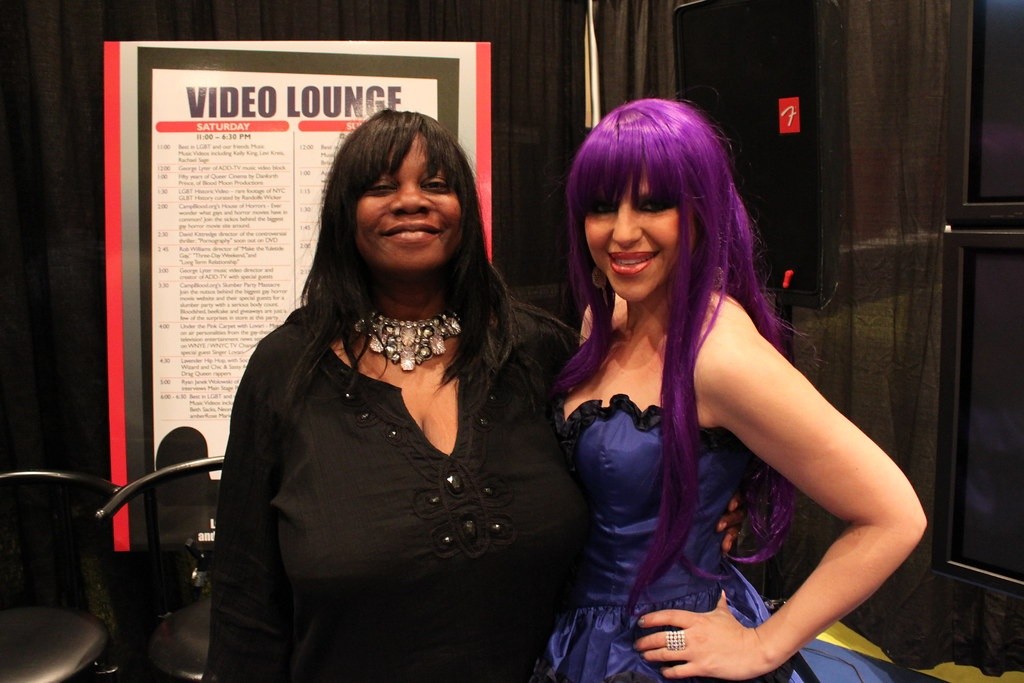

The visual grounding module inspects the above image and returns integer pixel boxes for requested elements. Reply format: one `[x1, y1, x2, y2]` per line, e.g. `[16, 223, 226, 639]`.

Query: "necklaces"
[354, 309, 462, 372]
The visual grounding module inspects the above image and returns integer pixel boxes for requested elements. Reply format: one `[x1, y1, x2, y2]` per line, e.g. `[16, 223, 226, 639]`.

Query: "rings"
[665, 630, 686, 651]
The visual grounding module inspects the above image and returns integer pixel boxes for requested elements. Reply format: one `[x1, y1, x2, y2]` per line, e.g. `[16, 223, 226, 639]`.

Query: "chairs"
[95, 457, 224, 683]
[0, 472, 124, 683]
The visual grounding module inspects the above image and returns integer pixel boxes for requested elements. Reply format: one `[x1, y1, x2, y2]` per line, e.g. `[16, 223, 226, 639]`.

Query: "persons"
[552, 97, 930, 683]
[198, 109, 742, 683]
